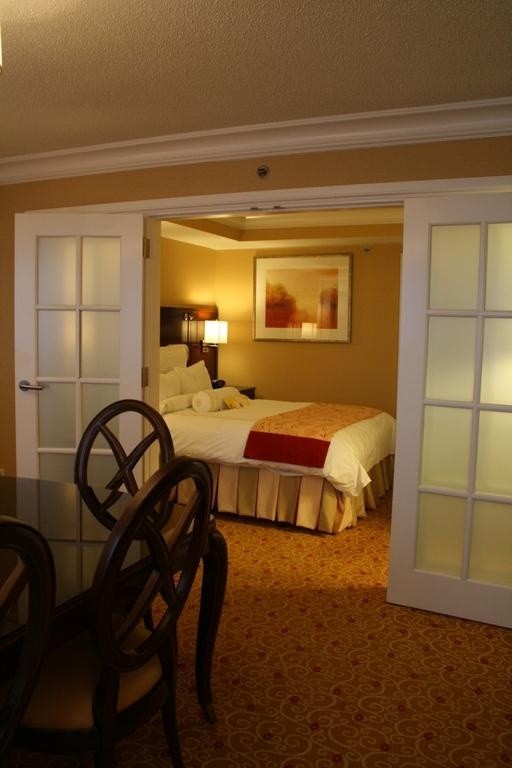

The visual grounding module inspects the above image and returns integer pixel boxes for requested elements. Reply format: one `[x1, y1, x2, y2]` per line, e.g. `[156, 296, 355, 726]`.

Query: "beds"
[152, 399, 397, 532]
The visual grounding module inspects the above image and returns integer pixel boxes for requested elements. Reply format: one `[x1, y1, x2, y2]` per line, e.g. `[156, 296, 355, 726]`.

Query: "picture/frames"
[253, 253, 353, 345]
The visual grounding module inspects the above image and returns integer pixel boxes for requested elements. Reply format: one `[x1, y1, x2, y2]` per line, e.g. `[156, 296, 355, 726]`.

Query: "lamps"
[202, 319, 228, 346]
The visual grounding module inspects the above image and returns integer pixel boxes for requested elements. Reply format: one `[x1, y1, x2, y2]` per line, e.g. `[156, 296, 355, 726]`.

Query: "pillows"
[158, 343, 240, 414]
